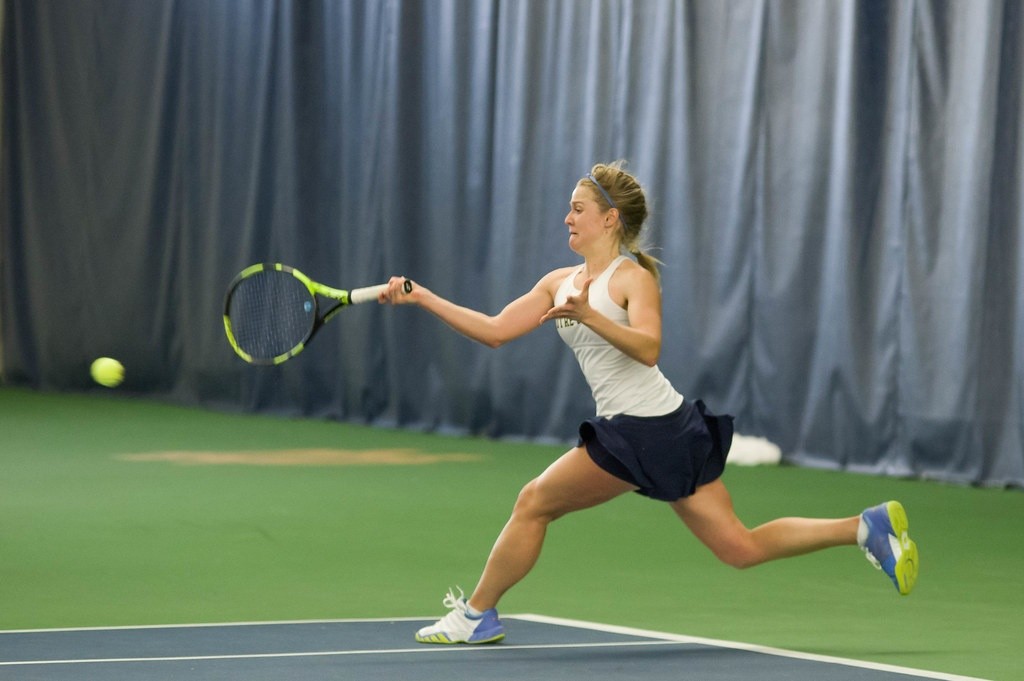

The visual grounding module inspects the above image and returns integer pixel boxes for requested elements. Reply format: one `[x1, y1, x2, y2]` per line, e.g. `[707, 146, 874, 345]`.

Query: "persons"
[373, 163, 922, 644]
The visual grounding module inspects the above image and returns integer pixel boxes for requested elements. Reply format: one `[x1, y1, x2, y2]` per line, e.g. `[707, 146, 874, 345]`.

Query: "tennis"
[91, 357, 124, 387]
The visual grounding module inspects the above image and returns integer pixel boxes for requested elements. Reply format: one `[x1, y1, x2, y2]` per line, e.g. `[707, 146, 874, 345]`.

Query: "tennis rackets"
[222, 263, 414, 365]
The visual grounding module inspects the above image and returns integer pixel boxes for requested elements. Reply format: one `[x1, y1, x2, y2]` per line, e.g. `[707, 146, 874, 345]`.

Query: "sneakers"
[857, 501, 919, 595]
[415, 597, 506, 644]
